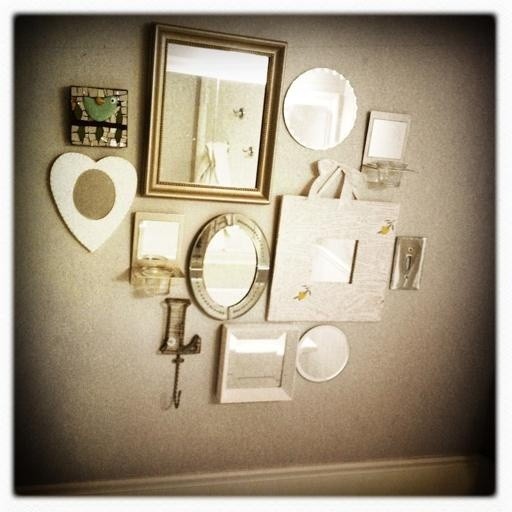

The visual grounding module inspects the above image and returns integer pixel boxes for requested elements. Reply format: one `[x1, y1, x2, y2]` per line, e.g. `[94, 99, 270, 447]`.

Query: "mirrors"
[185, 212, 271, 321]
[282, 67, 359, 152]
[296, 324, 351, 382]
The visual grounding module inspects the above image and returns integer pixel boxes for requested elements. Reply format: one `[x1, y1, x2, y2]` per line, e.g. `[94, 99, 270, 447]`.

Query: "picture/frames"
[362, 110, 412, 166]
[265, 193, 400, 322]
[48, 151, 139, 254]
[215, 318, 299, 404]
[140, 23, 288, 205]
[129, 210, 186, 271]
